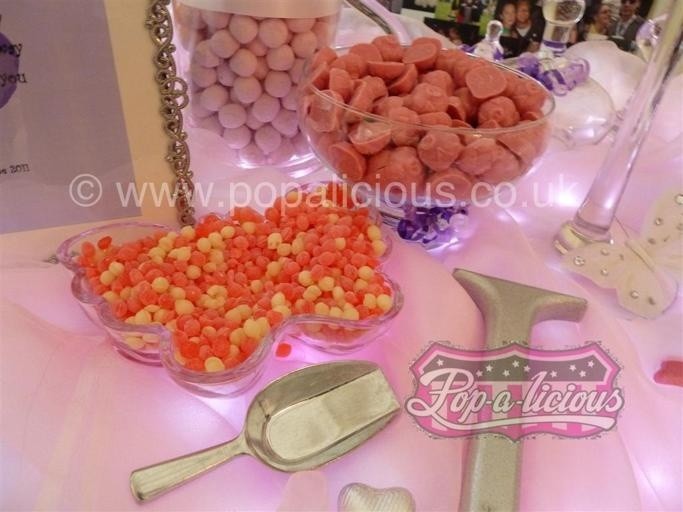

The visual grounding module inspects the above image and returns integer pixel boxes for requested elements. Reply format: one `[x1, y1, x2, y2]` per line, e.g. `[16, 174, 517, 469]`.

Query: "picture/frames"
[0, 0, 196, 264]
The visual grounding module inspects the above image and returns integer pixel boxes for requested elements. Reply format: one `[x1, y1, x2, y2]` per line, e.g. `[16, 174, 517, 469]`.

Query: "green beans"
[238, 310, 240, 311]
[76, 179, 392, 372]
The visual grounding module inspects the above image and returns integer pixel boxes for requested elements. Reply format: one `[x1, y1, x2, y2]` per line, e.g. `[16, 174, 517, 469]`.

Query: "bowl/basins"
[300, 44, 558, 228]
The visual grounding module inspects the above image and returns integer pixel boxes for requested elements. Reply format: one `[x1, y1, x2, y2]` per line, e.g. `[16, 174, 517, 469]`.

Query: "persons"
[610, 0, 665, 56]
[461, 1, 486, 22]
[430, 22, 447, 37]
[443, 22, 463, 47]
[494, 0, 520, 59]
[569, 3, 612, 45]
[512, 0, 545, 58]
[459, 0, 476, 21]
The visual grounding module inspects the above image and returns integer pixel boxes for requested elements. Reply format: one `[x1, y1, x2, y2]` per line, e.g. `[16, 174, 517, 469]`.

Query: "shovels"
[131, 360, 401, 505]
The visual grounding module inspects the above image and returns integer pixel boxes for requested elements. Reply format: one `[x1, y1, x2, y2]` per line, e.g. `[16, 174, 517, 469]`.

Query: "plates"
[55, 179, 405, 398]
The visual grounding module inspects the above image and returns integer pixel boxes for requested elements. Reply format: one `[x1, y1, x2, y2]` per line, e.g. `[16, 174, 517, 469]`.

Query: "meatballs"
[174, 3, 338, 166]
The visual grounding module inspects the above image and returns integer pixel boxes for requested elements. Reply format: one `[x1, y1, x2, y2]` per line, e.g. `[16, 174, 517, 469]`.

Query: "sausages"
[300, 36, 551, 206]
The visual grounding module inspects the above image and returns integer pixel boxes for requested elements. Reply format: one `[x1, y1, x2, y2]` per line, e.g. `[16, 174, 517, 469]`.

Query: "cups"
[525, 53, 622, 154]
